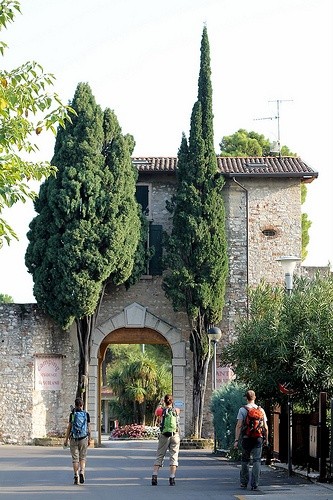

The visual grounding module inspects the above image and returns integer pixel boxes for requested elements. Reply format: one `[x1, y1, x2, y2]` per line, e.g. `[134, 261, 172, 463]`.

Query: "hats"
[243, 390, 255, 397]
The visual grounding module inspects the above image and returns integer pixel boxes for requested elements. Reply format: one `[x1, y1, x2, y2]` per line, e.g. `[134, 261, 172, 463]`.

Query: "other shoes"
[251, 486, 258, 490]
[80, 473, 85, 483]
[169, 477, 176, 486]
[240, 483, 248, 487]
[152, 475, 157, 486]
[74, 473, 79, 484]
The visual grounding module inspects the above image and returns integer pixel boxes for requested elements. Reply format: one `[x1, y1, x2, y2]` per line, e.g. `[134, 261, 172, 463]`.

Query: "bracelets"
[235, 439, 239, 442]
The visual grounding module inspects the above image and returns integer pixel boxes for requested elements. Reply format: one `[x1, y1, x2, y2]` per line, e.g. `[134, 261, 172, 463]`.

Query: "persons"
[64, 397, 91, 485]
[151, 394, 181, 486]
[233, 390, 269, 491]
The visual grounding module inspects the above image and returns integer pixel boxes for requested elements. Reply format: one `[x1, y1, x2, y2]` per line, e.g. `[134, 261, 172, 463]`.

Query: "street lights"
[207, 327, 223, 456]
[276, 255, 301, 486]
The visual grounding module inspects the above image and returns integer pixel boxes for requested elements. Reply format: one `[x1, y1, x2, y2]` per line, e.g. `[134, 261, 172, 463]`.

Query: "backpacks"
[72, 410, 88, 438]
[163, 409, 177, 432]
[243, 405, 264, 437]
